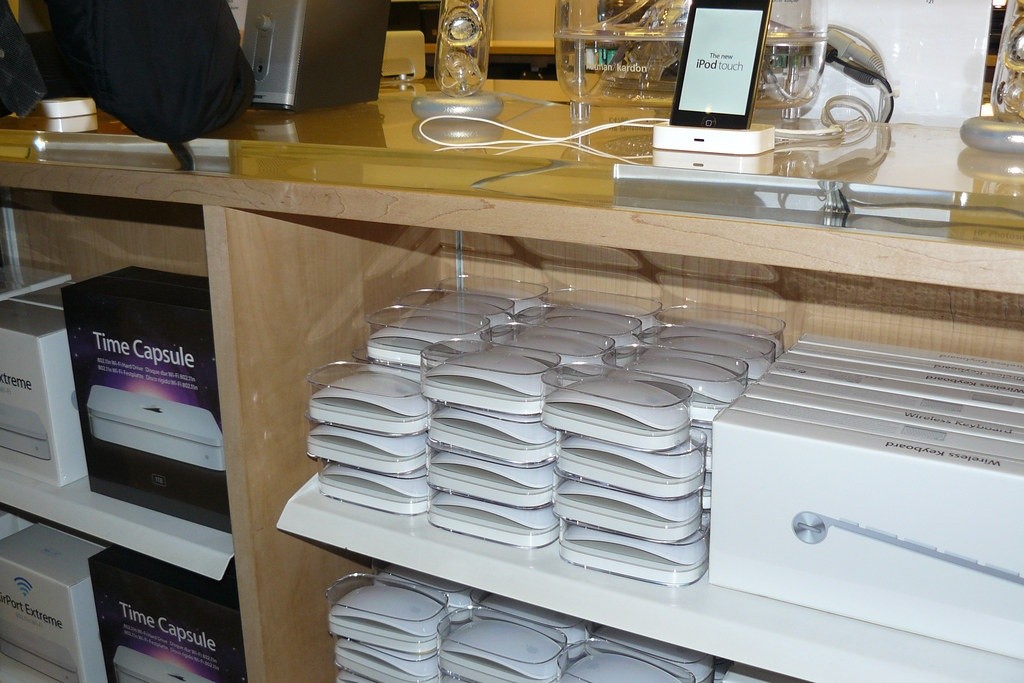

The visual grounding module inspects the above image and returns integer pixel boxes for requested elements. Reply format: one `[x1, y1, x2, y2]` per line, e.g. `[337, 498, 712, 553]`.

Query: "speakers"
[960, 0, 1024, 157]
[407, 0, 504, 120]
[241, 0, 392, 111]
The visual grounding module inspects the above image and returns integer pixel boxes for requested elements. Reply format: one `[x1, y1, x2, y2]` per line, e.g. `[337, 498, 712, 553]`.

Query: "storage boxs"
[0, 298, 88, 488]
[61, 265, 232, 536]
[0, 524, 108, 683]
[708, 333, 1024, 660]
[87, 544, 247, 683]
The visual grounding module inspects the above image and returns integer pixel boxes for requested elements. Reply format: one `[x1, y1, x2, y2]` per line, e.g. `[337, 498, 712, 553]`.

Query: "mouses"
[306, 292, 783, 683]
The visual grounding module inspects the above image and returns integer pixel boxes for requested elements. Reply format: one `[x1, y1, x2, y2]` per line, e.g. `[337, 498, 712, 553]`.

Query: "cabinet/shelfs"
[0, 78, 1024, 683]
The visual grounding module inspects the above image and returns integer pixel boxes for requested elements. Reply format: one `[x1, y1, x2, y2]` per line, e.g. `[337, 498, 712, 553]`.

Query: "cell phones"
[670, 0, 772, 130]
